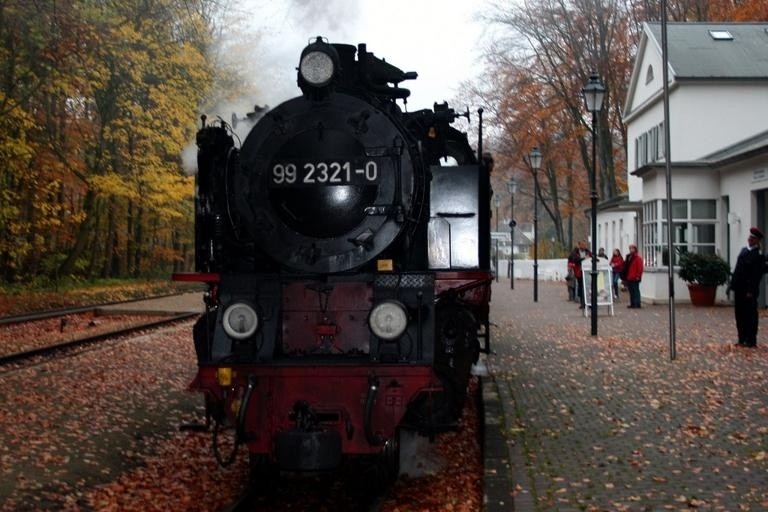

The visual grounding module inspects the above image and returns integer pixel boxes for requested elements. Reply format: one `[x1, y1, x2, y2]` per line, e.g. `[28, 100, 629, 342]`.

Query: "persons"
[598, 248, 608, 259]
[726, 227, 768, 348]
[624, 244, 643, 308]
[568, 239, 591, 309]
[609, 249, 625, 299]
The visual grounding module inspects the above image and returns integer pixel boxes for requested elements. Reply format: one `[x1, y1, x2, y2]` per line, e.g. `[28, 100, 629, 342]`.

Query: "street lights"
[507, 176, 519, 289]
[581, 68, 606, 337]
[528, 145, 544, 301]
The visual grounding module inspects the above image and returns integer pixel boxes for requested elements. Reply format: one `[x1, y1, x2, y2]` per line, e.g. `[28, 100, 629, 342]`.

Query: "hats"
[750, 227, 765, 239]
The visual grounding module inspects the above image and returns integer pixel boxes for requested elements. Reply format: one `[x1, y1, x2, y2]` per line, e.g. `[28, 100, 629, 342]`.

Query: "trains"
[172, 36, 496, 501]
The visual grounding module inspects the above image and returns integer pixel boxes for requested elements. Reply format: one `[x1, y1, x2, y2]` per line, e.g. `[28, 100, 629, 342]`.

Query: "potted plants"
[677, 249, 731, 305]
